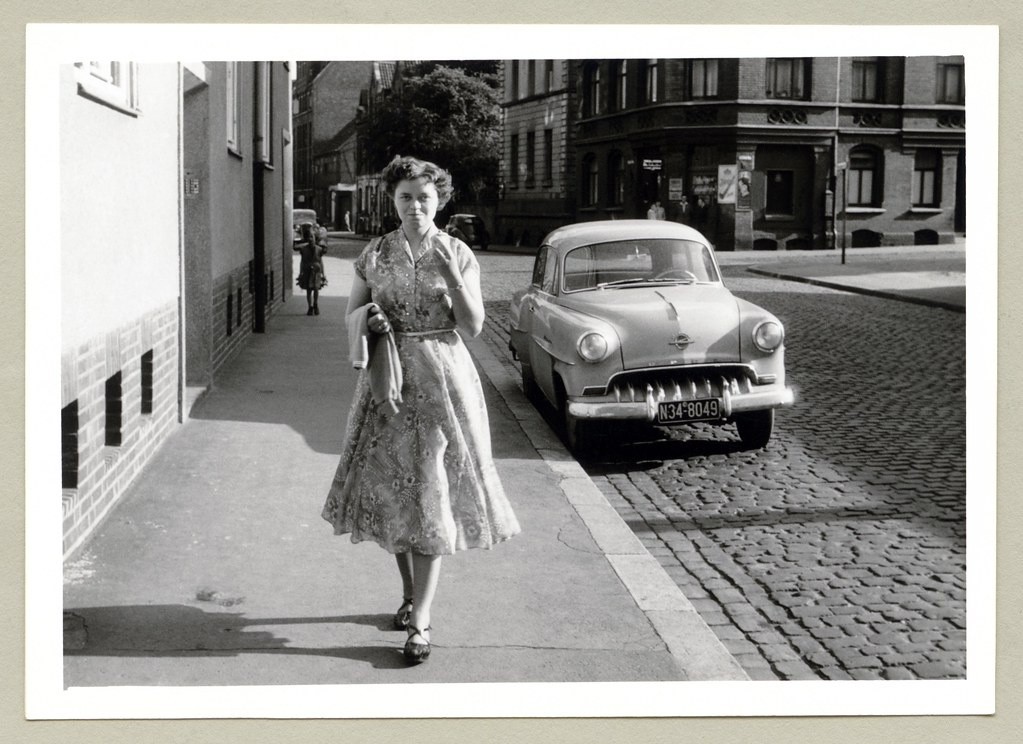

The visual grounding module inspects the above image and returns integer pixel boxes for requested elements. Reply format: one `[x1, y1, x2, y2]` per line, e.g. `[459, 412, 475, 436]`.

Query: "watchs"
[449, 282, 465, 291]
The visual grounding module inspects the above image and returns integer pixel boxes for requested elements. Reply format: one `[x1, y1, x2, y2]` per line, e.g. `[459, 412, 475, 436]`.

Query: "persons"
[647, 204, 656, 220]
[293, 226, 328, 315]
[677, 195, 693, 225]
[321, 156, 521, 662]
[655, 198, 665, 220]
[698, 197, 710, 234]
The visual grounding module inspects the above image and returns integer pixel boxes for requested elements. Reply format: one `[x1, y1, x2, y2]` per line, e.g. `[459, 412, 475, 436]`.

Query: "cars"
[438, 214, 490, 252]
[293, 210, 319, 245]
[505, 218, 794, 449]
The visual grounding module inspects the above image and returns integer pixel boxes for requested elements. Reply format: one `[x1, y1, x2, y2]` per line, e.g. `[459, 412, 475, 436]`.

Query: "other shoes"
[403, 623, 433, 663]
[313, 304, 319, 315]
[307, 306, 313, 316]
[394, 599, 413, 630]
